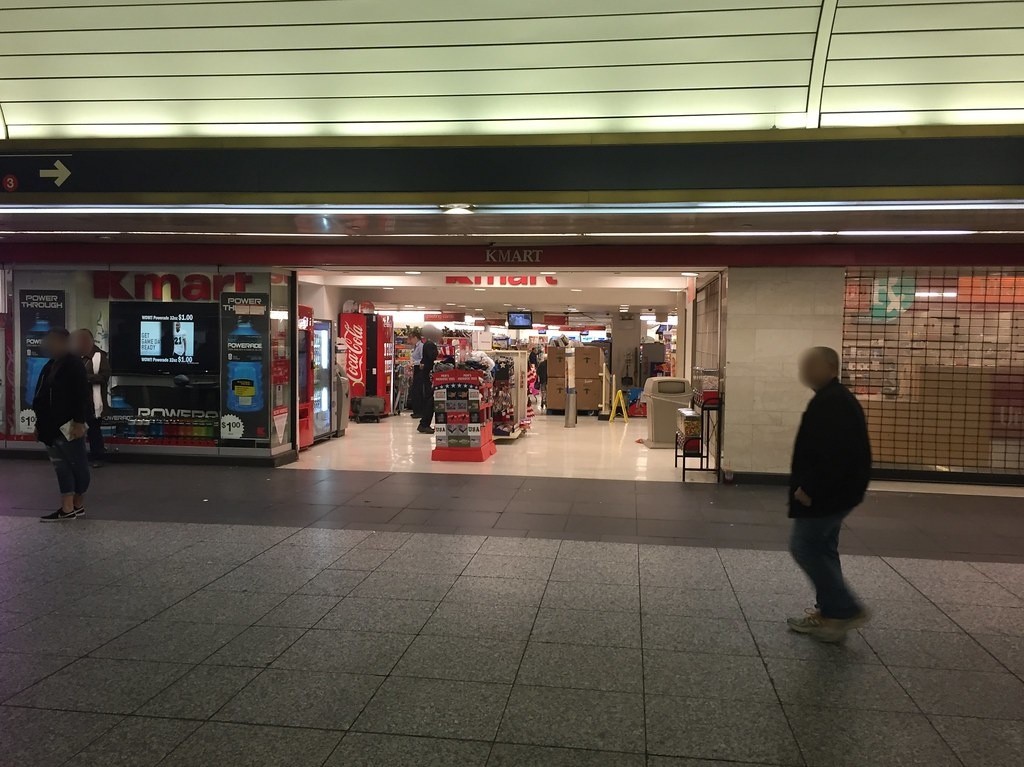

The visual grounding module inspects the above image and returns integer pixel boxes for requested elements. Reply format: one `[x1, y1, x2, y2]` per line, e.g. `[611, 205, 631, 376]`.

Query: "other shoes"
[410, 412, 422, 418]
[417, 424, 436, 434]
[91, 459, 106, 468]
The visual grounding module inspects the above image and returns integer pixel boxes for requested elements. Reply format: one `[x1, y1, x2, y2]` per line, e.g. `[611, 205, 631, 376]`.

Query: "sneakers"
[40, 508, 77, 522]
[809, 605, 872, 644]
[787, 609, 834, 632]
[72, 505, 86, 516]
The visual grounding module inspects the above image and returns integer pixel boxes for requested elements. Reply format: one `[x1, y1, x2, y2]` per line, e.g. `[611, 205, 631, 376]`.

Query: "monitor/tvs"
[108, 300, 220, 377]
[655, 325, 673, 334]
[507, 312, 533, 329]
[580, 331, 589, 335]
[538, 330, 546, 334]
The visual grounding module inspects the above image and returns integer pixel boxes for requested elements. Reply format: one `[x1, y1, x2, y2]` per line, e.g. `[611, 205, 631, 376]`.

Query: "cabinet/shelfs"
[394, 345, 456, 416]
[675, 391, 723, 483]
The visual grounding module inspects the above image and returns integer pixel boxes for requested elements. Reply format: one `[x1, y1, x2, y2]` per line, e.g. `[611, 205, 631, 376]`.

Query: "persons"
[79, 329, 110, 468]
[787, 347, 875, 643]
[407, 330, 424, 419]
[417, 334, 439, 435]
[529, 347, 539, 390]
[536, 354, 547, 409]
[33, 327, 90, 522]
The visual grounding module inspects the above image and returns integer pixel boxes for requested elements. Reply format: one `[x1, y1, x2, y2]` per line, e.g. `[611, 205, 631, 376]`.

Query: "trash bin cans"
[644, 377, 693, 449]
[333, 362, 351, 438]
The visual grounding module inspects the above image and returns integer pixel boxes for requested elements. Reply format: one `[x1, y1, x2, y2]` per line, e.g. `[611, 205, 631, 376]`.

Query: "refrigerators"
[314, 319, 337, 445]
[338, 312, 394, 421]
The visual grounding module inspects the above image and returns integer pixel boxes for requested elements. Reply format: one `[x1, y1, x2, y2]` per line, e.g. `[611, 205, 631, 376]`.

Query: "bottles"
[23, 311, 52, 406]
[226, 315, 264, 412]
[116, 412, 219, 440]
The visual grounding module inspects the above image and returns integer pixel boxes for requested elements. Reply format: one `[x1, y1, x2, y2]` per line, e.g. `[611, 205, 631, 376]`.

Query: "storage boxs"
[547, 345, 605, 379]
[677, 390, 720, 453]
[548, 379, 602, 410]
